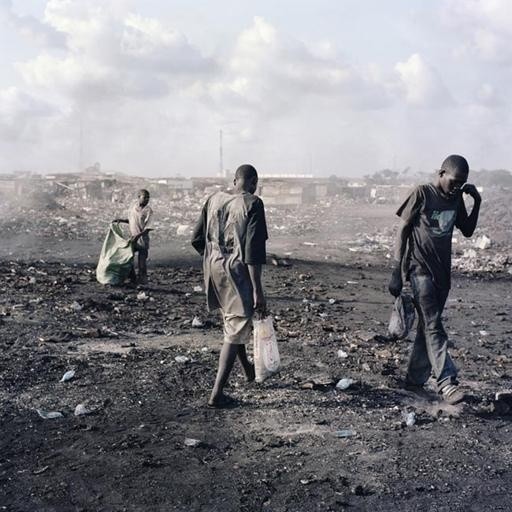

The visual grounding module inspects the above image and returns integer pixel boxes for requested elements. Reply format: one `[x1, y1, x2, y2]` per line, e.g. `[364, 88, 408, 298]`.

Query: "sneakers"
[404, 380, 467, 405]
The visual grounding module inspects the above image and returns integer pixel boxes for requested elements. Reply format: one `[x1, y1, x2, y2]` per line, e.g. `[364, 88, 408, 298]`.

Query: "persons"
[111, 189, 155, 289]
[388, 154, 482, 405]
[189, 165, 269, 408]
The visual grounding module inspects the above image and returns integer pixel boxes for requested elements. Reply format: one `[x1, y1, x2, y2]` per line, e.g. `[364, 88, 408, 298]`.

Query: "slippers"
[207, 396, 237, 408]
[246, 364, 255, 382]
